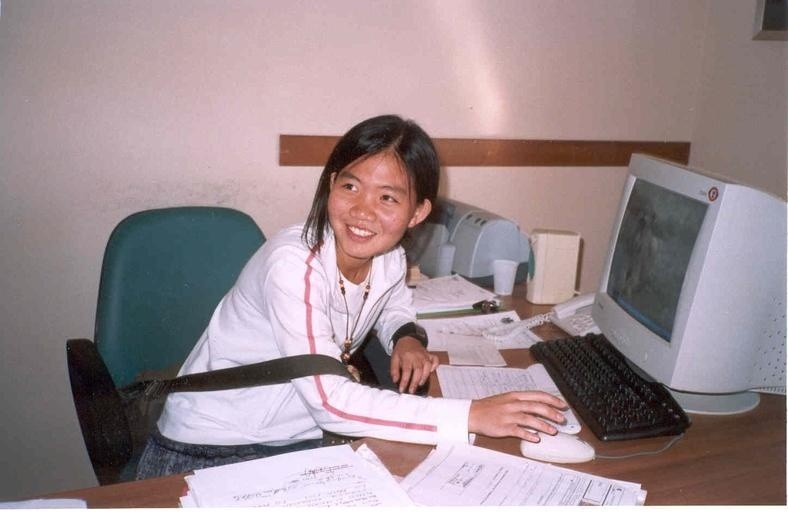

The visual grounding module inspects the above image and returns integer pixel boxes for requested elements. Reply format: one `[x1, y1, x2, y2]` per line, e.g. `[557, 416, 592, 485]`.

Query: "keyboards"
[530, 334, 691, 441]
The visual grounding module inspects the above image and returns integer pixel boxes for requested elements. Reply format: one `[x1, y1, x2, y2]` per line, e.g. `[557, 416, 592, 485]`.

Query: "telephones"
[549, 291, 602, 337]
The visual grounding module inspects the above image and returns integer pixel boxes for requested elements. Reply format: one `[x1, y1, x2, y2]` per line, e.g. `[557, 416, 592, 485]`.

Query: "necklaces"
[336, 259, 371, 383]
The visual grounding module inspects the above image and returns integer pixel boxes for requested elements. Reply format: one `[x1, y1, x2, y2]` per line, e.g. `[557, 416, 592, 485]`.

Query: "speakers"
[526, 228, 582, 305]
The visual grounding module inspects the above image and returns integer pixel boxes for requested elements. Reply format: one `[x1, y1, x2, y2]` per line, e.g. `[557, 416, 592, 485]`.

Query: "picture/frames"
[753, 1, 788, 42]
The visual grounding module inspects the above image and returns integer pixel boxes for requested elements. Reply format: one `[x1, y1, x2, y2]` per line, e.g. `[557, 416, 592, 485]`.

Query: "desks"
[1, 281, 786, 507]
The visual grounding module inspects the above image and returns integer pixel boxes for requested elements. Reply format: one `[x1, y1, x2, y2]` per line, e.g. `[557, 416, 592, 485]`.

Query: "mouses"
[520, 432, 595, 463]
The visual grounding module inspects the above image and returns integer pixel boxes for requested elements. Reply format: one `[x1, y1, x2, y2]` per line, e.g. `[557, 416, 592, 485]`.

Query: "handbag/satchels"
[62, 335, 138, 485]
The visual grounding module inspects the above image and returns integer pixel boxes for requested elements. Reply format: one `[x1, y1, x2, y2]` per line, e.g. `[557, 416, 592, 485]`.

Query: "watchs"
[393, 323, 428, 349]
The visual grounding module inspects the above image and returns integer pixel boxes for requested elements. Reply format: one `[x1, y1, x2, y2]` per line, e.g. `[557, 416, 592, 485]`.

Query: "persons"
[131, 119, 568, 481]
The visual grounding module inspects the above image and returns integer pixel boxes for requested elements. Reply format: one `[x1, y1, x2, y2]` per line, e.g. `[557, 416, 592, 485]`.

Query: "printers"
[402, 195, 530, 288]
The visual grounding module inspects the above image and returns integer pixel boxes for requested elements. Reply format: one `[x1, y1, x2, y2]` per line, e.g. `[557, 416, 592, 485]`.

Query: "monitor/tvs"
[590, 153, 787, 417]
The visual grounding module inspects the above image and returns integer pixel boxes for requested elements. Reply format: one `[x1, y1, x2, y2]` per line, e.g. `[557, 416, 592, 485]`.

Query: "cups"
[493, 259, 518, 296]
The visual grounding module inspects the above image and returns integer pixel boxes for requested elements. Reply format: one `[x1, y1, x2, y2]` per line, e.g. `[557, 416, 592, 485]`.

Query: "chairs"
[59, 202, 385, 488]
